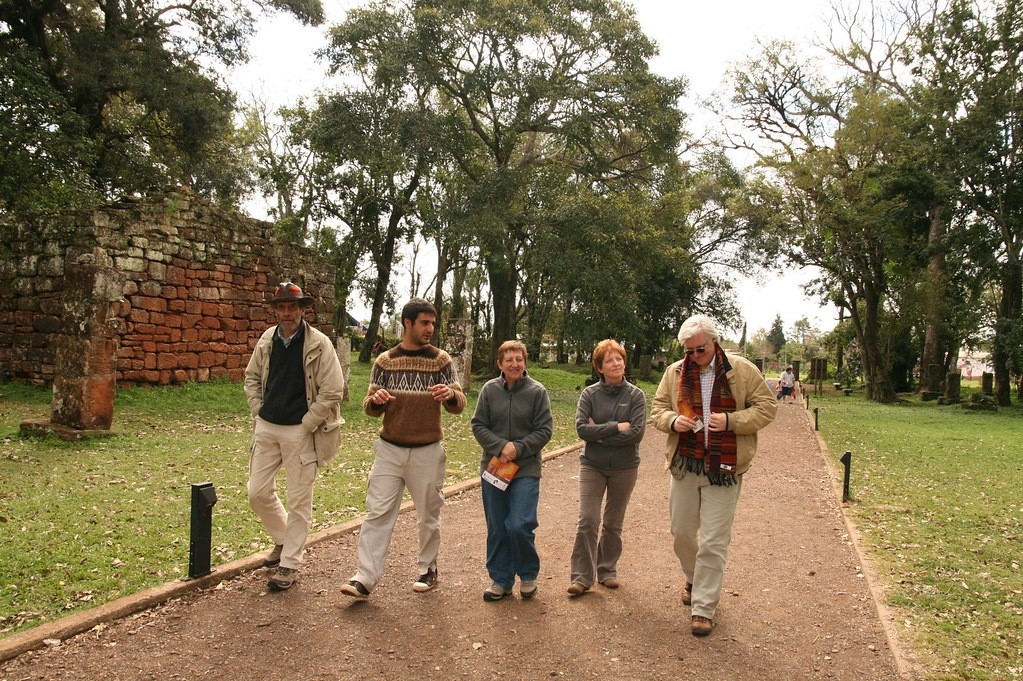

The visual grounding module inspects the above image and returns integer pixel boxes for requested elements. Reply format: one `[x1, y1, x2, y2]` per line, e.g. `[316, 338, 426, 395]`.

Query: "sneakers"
[681, 581, 693, 605]
[412, 567, 439, 592]
[264, 544, 283, 568]
[691, 615, 713, 635]
[341, 580, 371, 599]
[603, 577, 619, 588]
[482, 583, 513, 601]
[519, 579, 539, 600]
[567, 583, 586, 594]
[266, 567, 301, 589]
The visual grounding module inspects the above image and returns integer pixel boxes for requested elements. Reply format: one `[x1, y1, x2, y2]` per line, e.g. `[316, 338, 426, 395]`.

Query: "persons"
[776, 367, 795, 404]
[244, 282, 345, 590]
[342, 298, 467, 599]
[650, 313, 779, 634]
[470, 341, 553, 600]
[567, 339, 646, 596]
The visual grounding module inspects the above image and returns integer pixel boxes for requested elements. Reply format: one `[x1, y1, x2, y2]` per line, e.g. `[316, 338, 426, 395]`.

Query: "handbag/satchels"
[793, 390, 797, 399]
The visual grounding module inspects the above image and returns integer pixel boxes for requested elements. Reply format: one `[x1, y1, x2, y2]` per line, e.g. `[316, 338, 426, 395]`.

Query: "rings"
[446, 396, 450, 400]
[682, 425, 684, 429]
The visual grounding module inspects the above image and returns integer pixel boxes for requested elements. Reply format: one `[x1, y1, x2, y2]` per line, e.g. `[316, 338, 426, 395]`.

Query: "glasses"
[683, 340, 710, 355]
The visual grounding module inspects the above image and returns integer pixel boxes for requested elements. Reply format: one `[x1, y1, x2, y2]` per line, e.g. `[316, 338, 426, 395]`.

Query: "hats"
[269, 282, 314, 308]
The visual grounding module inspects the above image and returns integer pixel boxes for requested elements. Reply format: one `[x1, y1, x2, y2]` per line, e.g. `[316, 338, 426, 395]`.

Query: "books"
[482, 456, 519, 491]
